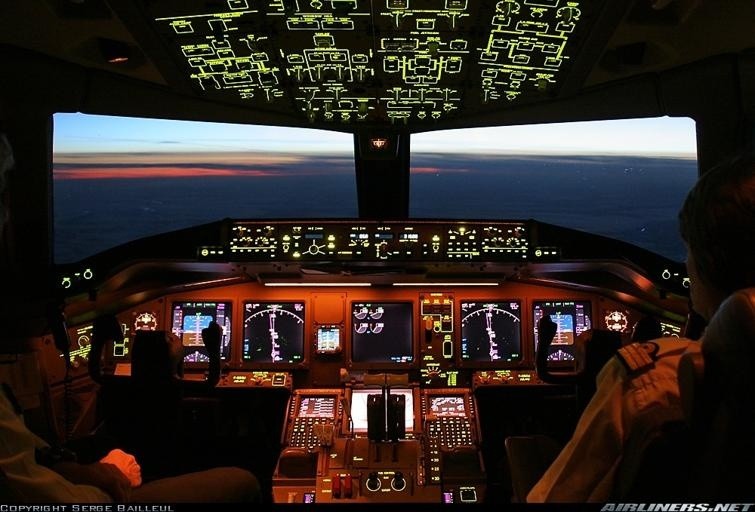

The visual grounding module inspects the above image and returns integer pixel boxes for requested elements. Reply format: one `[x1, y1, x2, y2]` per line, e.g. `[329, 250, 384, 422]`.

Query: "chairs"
[588, 285, 754, 511]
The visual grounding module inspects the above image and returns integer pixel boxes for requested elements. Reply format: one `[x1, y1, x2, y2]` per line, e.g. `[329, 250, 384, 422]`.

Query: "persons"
[0, 381, 267, 505]
[523, 142, 754, 506]
